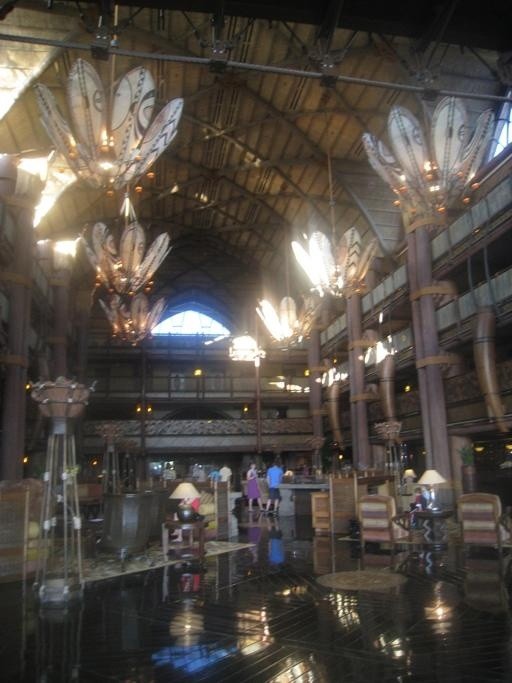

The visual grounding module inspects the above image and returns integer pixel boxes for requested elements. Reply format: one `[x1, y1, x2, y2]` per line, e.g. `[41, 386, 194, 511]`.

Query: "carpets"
[63, 538, 256, 584]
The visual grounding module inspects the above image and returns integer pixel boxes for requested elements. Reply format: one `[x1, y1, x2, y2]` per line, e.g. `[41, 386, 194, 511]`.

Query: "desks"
[274, 479, 330, 516]
[56, 515, 104, 560]
[414, 503, 453, 550]
[162, 519, 207, 559]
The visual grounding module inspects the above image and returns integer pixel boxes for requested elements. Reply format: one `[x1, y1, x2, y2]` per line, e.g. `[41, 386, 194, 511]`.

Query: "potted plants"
[458, 442, 479, 493]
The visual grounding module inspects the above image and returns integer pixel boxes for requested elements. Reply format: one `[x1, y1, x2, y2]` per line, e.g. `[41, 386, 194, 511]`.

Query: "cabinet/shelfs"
[312, 535, 352, 573]
[309, 489, 353, 536]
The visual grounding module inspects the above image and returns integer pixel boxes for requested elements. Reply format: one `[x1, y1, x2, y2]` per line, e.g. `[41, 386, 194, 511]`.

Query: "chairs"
[356, 495, 414, 565]
[93, 493, 158, 570]
[459, 551, 512, 616]
[147, 486, 180, 535]
[450, 493, 511, 560]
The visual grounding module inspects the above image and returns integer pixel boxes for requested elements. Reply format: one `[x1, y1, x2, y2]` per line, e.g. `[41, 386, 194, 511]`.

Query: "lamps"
[96, 288, 166, 345]
[373, 418, 405, 488]
[79, 179, 174, 299]
[34, 0, 183, 195]
[290, 38, 375, 298]
[24, 371, 97, 604]
[256, 196, 319, 351]
[227, 283, 266, 364]
[268, 433, 328, 473]
[418, 469, 446, 511]
[97, 418, 138, 493]
[168, 482, 203, 521]
[360, 1, 497, 226]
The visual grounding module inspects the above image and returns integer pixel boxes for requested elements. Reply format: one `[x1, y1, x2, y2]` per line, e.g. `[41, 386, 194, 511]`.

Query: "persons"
[409, 485, 431, 523]
[265, 461, 284, 512]
[208, 463, 233, 487]
[246, 464, 266, 512]
[169, 497, 204, 543]
